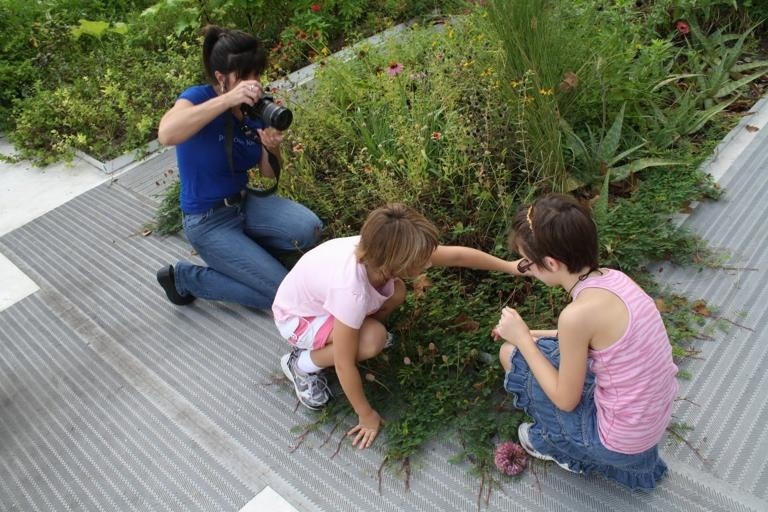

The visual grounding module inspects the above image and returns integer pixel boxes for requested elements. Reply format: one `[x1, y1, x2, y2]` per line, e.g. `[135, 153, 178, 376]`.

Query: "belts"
[214, 188, 247, 210]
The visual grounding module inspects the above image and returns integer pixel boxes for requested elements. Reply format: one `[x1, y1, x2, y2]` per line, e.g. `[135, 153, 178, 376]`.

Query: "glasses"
[518, 258, 546, 274]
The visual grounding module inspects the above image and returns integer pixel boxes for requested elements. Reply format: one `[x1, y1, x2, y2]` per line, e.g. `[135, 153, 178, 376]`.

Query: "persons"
[490, 192, 679, 489]
[270, 204, 534, 450]
[156, 25, 324, 310]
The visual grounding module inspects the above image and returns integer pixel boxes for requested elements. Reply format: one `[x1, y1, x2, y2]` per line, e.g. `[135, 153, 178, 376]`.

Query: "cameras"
[240, 92, 293, 132]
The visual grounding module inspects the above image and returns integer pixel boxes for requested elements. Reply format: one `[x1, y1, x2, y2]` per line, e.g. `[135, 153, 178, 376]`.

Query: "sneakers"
[281, 349, 330, 409]
[518, 421, 583, 475]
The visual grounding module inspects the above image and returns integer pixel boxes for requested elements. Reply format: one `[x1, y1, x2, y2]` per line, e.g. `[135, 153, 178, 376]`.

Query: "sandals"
[158, 265, 197, 306]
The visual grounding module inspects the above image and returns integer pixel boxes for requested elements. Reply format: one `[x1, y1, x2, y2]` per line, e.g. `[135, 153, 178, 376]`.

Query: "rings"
[249, 84, 255, 92]
